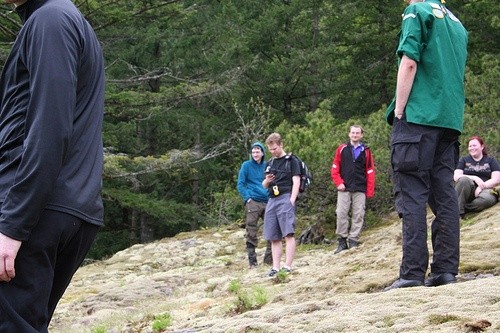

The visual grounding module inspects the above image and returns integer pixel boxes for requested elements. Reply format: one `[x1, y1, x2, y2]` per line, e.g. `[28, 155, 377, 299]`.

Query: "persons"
[386, 0, 467, 290]
[331, 124, 375, 255]
[237, 142, 276, 268]
[0, 0, 107, 333]
[451, 136, 500, 222]
[262, 132, 302, 277]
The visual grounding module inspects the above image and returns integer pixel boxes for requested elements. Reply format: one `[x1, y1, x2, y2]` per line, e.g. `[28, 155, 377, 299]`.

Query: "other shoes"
[281, 268, 290, 274]
[424, 272, 458, 287]
[268, 269, 278, 276]
[383, 278, 424, 291]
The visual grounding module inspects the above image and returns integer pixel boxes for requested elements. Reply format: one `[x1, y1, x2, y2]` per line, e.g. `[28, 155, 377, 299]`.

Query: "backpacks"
[269, 152, 311, 199]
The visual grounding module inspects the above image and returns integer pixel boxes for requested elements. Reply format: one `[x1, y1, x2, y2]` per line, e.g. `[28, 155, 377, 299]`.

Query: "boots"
[349, 239, 359, 248]
[334, 239, 347, 254]
